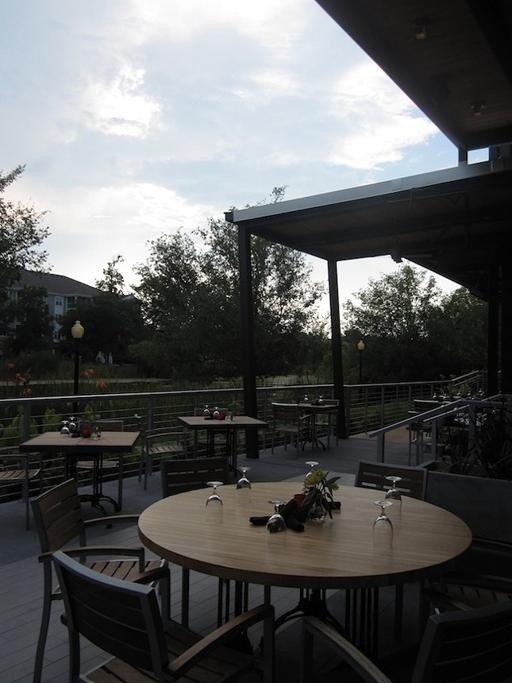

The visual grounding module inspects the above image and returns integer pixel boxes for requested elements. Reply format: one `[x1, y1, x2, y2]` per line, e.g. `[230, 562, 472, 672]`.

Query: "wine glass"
[371, 476, 402, 539]
[236, 467, 251, 493]
[204, 481, 223, 514]
[59, 417, 75, 435]
[304, 460, 319, 489]
[265, 499, 287, 535]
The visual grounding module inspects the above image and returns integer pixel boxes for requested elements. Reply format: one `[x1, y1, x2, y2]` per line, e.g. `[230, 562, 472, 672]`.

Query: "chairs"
[160, 459, 244, 500]
[271, 403, 310, 455]
[298, 603, 509, 683]
[193, 408, 234, 450]
[32, 480, 170, 682]
[405, 399, 510, 468]
[74, 421, 123, 495]
[1, 468, 42, 529]
[52, 551, 271, 683]
[133, 413, 190, 488]
[315, 400, 339, 446]
[354, 461, 428, 615]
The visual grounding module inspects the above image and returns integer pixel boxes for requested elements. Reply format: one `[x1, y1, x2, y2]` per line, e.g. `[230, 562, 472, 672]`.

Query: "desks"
[19, 431, 142, 529]
[178, 417, 266, 470]
[139, 481, 472, 676]
[299, 404, 337, 451]
